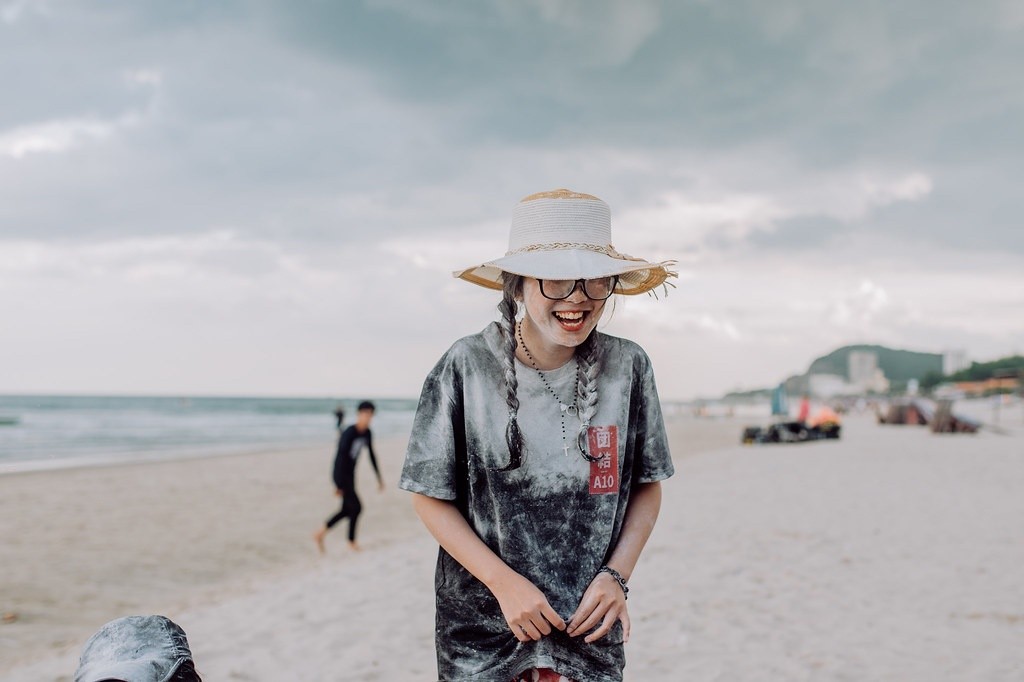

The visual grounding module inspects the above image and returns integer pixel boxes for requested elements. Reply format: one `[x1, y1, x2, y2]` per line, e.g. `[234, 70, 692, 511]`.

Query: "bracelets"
[599, 565, 629, 601]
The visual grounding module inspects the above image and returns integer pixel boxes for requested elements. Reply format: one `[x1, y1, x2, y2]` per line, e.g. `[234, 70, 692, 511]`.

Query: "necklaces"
[518, 317, 580, 457]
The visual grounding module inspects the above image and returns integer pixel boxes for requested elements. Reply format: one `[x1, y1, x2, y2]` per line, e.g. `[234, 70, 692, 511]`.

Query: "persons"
[73, 615, 204, 682]
[335, 409, 344, 428]
[397, 189, 678, 682]
[313, 401, 384, 554]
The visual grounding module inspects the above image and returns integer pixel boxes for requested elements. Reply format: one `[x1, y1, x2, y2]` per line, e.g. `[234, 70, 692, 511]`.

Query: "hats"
[73, 615, 194, 682]
[452, 189, 678, 300]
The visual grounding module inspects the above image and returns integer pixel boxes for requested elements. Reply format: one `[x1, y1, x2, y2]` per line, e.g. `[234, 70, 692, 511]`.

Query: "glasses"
[535, 276, 621, 300]
